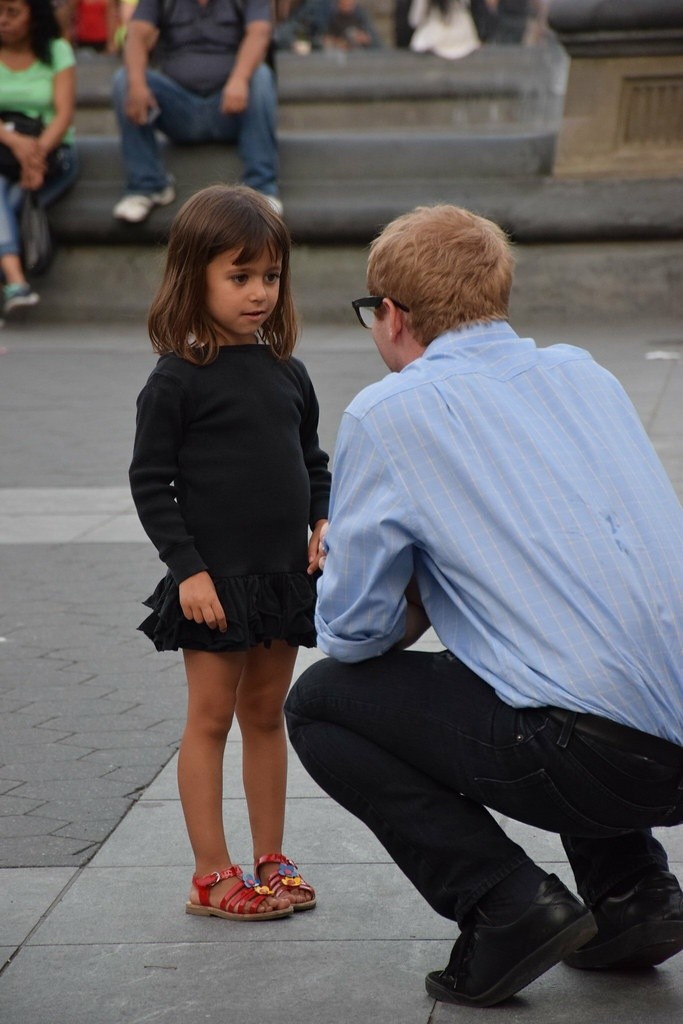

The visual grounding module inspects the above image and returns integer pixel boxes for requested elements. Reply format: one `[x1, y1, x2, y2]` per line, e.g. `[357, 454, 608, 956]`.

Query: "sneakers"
[113, 186, 175, 222]
[559, 871, 683, 971]
[425, 872, 597, 1007]
[268, 195, 283, 216]
[3, 280, 39, 314]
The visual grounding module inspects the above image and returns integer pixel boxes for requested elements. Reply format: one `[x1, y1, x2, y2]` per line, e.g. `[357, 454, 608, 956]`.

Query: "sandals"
[253, 853, 317, 910]
[186, 865, 294, 920]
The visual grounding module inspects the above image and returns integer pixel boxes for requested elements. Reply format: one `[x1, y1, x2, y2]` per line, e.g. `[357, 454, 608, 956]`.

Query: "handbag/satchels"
[0, 110, 71, 186]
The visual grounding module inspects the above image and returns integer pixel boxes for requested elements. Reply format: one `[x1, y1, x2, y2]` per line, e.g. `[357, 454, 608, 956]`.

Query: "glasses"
[351, 296, 412, 329]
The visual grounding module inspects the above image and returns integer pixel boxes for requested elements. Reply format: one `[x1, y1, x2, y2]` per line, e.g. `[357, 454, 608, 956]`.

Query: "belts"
[549, 708, 683, 769]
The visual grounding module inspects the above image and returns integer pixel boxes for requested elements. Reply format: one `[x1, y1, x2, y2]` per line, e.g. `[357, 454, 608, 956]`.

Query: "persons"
[0, 0, 544, 320]
[129, 186, 333, 922]
[281, 207, 681, 1011]
